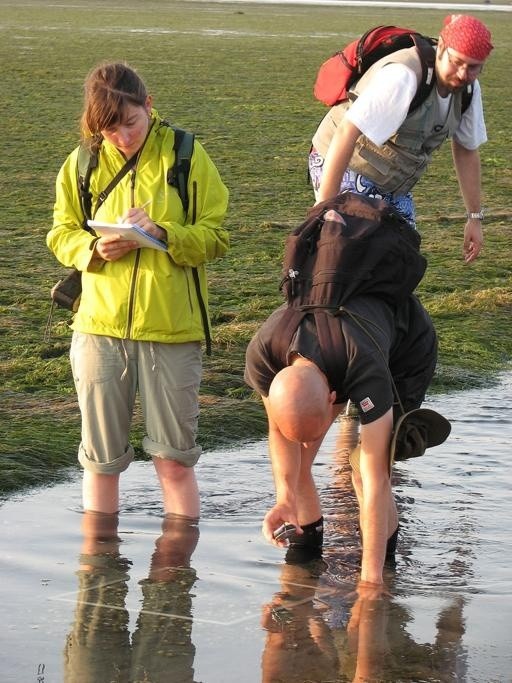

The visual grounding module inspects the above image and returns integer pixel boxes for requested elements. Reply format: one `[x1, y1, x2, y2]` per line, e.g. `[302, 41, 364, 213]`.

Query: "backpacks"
[313, 26, 473, 114]
[270, 192, 427, 389]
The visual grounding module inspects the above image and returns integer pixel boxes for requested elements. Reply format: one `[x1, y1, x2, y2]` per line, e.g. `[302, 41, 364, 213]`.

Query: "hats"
[348, 409, 451, 479]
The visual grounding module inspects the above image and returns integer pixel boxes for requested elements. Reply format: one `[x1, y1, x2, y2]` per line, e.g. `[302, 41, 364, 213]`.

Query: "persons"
[46, 65, 229, 518]
[242, 290, 437, 585]
[63, 518, 199, 683]
[309, 13, 495, 263]
[262, 563, 466, 683]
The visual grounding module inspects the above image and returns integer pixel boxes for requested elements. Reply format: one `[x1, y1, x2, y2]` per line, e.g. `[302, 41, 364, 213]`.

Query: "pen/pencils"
[121, 200, 151, 225]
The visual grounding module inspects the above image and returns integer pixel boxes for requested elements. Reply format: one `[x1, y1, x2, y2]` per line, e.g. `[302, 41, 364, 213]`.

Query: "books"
[86, 220, 167, 253]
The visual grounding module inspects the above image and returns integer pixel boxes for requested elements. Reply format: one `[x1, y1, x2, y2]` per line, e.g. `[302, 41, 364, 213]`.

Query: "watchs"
[466, 210, 485, 220]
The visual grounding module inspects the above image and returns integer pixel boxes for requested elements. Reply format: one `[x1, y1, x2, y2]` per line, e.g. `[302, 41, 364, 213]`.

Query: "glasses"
[447, 47, 482, 75]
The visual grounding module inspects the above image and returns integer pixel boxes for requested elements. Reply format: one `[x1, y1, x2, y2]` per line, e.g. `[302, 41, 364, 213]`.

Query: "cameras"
[274, 518, 298, 544]
